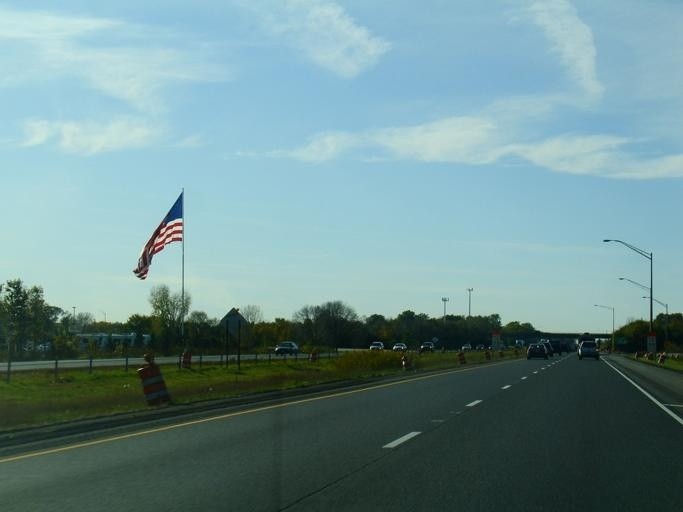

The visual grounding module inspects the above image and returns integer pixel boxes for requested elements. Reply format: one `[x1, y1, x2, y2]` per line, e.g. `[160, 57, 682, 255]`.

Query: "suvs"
[577, 334, 599, 360]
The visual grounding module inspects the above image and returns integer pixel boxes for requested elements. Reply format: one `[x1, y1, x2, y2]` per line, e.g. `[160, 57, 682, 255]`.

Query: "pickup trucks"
[421, 342, 437, 351]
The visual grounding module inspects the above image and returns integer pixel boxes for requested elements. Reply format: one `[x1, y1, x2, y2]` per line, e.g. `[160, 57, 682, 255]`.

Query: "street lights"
[603, 240, 668, 353]
[467, 288, 473, 319]
[442, 297, 449, 326]
[594, 304, 616, 353]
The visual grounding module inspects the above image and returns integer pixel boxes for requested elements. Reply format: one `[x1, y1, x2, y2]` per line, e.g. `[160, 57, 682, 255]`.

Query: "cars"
[463, 343, 496, 351]
[527, 340, 576, 360]
[275, 342, 298, 355]
[393, 343, 407, 351]
[370, 341, 384, 351]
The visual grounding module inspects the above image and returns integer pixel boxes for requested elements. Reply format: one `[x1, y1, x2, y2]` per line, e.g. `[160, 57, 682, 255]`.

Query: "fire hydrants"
[401, 356, 407, 368]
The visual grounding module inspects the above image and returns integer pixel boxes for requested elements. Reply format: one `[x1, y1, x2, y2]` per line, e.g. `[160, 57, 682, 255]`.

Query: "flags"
[135, 190, 182, 279]
